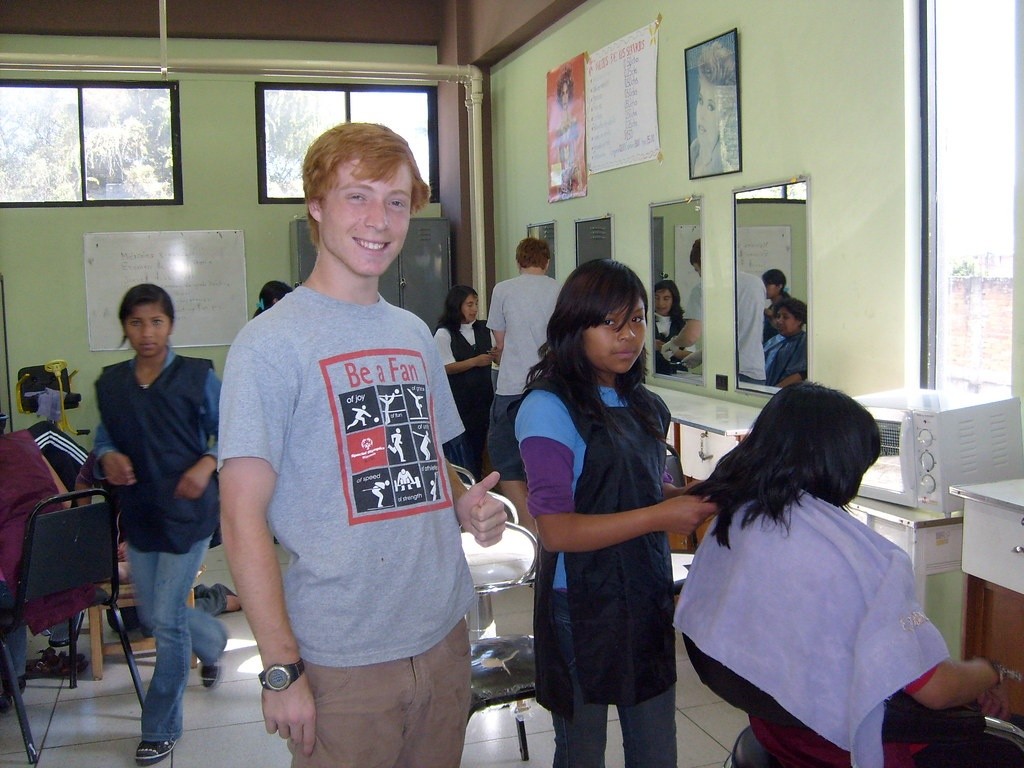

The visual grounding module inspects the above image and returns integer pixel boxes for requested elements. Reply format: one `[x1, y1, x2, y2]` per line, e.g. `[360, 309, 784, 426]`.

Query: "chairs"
[681, 630, 1024, 768]
[86, 561, 206, 684]
[0, 488, 146, 768]
[444, 462, 541, 762]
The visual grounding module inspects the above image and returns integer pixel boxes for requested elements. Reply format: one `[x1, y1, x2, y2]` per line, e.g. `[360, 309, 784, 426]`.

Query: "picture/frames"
[684, 27, 741, 182]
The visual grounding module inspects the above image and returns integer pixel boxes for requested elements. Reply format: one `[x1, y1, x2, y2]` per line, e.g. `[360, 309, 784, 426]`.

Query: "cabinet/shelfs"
[291, 219, 451, 334]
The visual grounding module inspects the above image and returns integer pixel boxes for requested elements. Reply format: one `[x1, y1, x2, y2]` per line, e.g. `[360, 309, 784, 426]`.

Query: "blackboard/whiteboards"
[83, 229, 250, 351]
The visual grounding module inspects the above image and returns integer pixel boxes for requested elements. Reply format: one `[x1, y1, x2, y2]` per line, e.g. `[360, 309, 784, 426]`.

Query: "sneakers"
[135, 740, 178, 766]
[34, 647, 88, 674]
[200, 638, 228, 687]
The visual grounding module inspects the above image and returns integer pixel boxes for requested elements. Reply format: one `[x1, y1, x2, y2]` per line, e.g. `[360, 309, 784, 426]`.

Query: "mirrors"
[576, 214, 616, 268]
[648, 193, 705, 388]
[734, 176, 815, 393]
[525, 220, 558, 277]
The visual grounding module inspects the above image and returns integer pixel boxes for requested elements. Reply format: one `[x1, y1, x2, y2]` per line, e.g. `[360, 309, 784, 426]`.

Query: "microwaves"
[851, 387, 1024, 516]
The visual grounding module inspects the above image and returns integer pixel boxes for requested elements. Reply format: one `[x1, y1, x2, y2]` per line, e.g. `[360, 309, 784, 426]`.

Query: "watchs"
[258, 657, 305, 691]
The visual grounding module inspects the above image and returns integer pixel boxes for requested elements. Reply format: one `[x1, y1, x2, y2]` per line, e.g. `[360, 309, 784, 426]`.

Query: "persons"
[654, 238, 808, 389]
[509, 258, 720, 768]
[0, 421, 242, 712]
[91, 282, 230, 766]
[684, 380, 1023, 768]
[249, 237, 565, 541]
[217, 123, 508, 768]
